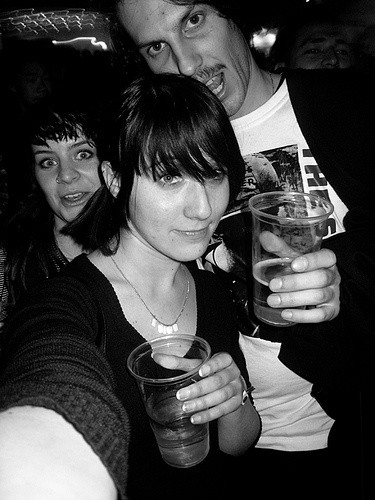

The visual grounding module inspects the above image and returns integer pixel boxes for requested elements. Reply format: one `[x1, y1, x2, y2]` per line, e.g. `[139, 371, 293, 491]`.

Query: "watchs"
[240, 375, 249, 407]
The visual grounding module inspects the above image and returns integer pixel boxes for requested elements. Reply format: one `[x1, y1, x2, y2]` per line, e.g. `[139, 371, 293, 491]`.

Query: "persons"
[277, 9, 360, 69]
[0, 91, 126, 332]
[0, 60, 56, 107]
[0, 73, 261, 500]
[115, 0, 375, 499]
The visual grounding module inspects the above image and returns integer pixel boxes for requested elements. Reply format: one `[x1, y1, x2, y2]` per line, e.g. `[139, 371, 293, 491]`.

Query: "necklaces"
[268, 72, 276, 93]
[110, 253, 190, 326]
